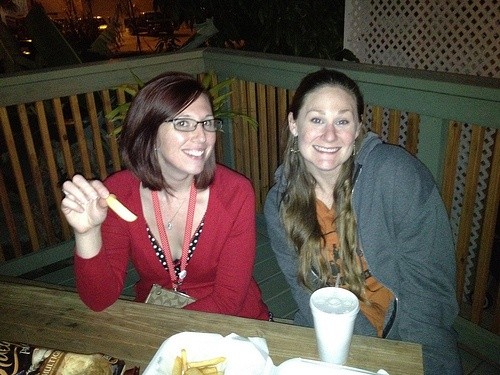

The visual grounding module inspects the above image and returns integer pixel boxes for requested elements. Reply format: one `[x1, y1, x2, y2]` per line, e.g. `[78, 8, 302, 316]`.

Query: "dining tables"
[0, 275, 423, 375]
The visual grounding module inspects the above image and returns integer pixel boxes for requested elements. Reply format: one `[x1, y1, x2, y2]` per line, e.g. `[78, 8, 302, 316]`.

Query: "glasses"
[163, 117, 224, 132]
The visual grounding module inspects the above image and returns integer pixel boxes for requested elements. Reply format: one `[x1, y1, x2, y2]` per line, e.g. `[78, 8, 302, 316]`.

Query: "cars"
[124, 11, 174, 36]
[78, 17, 109, 36]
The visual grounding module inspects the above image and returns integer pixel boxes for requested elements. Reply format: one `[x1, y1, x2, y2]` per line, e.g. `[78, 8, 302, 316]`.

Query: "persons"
[61, 71, 274, 322]
[264, 70, 463, 375]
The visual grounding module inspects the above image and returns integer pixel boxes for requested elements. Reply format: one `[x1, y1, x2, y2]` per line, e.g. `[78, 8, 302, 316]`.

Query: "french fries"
[171, 349, 225, 375]
[106, 196, 138, 222]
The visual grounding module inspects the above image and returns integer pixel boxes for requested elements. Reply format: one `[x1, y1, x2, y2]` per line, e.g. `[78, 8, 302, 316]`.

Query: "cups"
[309, 286, 361, 364]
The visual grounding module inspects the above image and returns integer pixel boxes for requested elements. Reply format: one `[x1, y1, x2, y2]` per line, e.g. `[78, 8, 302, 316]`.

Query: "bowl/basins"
[142, 329, 379, 375]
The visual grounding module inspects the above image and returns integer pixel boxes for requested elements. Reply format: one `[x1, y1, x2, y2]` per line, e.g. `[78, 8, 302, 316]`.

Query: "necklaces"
[166, 198, 186, 229]
